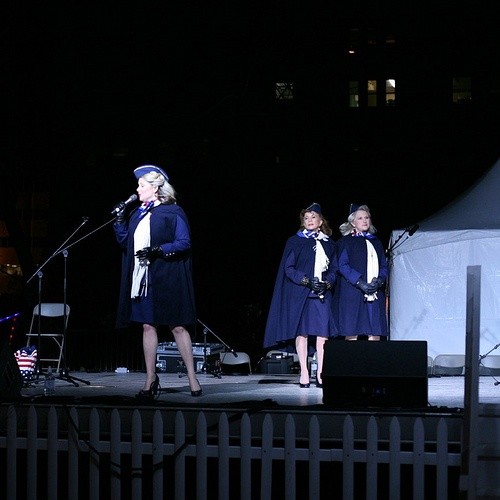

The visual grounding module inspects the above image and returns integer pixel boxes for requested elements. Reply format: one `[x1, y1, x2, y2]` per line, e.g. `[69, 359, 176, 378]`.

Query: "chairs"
[479, 355, 500, 376]
[434, 354, 466, 376]
[25, 303, 70, 373]
[220, 352, 253, 375]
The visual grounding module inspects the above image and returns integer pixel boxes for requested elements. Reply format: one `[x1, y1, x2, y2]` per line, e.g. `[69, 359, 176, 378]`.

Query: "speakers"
[0, 330, 23, 398]
[321, 340, 428, 409]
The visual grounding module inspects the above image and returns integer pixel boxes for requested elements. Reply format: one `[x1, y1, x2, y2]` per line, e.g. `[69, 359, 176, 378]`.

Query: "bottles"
[310, 355, 318, 378]
[117, 368, 129, 373]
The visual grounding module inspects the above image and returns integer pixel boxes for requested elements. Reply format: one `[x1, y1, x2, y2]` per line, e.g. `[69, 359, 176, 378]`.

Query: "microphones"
[110, 194, 137, 214]
[312, 277, 324, 303]
[228, 347, 237, 357]
[364, 277, 377, 301]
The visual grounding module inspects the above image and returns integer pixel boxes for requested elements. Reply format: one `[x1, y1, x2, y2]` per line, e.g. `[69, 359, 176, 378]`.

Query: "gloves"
[115, 202, 126, 223]
[356, 279, 375, 295]
[134, 246, 161, 260]
[368, 277, 384, 293]
[302, 277, 331, 295]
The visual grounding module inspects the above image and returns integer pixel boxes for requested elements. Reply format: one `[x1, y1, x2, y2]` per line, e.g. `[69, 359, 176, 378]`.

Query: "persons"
[336, 204, 388, 340]
[284, 202, 338, 388]
[114, 165, 203, 397]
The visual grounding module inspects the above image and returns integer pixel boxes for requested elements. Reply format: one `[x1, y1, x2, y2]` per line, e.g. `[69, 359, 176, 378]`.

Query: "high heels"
[135, 372, 159, 399]
[316, 375, 322, 388]
[299, 375, 310, 388]
[189, 378, 202, 397]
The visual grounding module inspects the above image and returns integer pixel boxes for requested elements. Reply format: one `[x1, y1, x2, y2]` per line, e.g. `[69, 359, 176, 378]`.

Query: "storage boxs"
[155, 342, 225, 374]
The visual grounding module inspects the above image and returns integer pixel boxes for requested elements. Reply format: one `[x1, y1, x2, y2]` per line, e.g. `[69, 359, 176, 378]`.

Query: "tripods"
[179, 319, 222, 378]
[23, 206, 127, 387]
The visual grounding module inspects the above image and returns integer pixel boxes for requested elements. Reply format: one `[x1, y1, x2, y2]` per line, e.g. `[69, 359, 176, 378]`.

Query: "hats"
[349, 203, 362, 214]
[134, 165, 169, 182]
[307, 203, 321, 214]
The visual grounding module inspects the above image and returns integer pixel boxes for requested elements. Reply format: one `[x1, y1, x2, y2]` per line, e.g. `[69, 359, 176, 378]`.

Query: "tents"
[391, 164, 500, 360]
